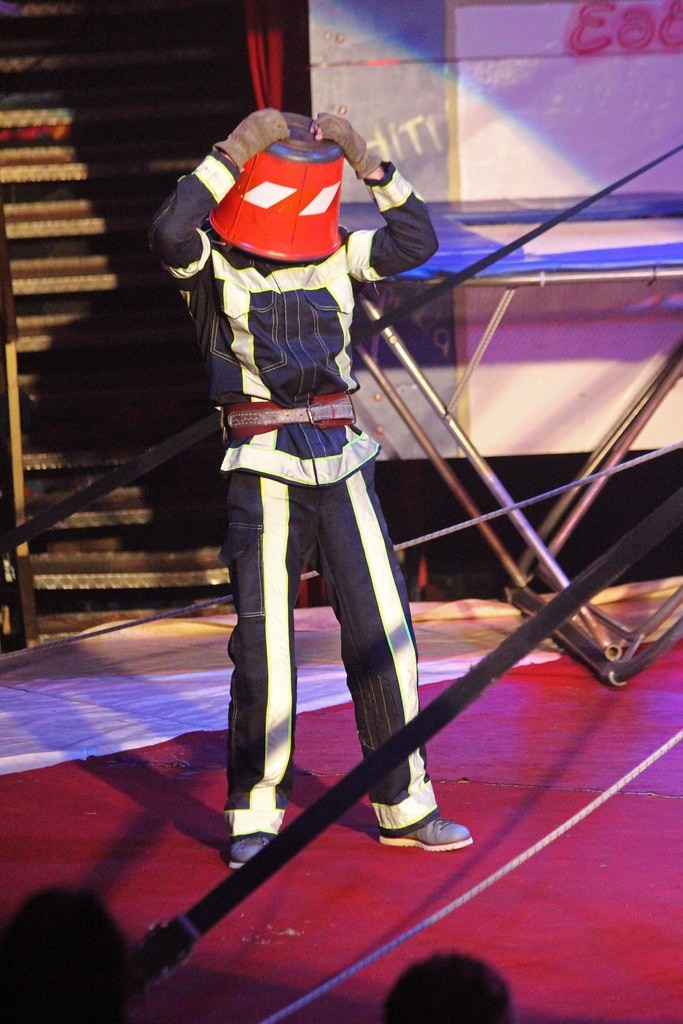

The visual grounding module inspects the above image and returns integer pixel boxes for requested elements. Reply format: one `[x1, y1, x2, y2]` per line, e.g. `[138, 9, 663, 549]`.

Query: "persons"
[149, 108, 473, 869]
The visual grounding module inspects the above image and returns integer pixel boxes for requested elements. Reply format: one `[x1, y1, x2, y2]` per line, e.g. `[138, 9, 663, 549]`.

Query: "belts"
[227, 398, 354, 427]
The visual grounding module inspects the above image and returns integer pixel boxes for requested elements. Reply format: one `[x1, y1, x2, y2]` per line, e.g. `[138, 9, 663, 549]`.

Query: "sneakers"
[380, 818, 474, 851]
[229, 837, 266, 869]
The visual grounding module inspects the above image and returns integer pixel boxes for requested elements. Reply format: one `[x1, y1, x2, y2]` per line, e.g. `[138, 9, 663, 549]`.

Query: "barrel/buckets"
[208, 112, 344, 262]
